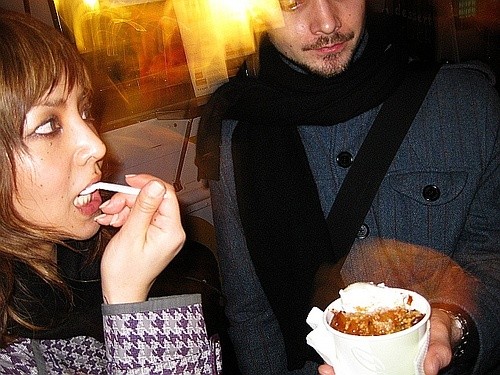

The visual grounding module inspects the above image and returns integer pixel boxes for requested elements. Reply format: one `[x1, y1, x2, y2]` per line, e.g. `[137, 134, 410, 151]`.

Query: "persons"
[0, 6, 224, 375]
[207, 0, 500, 375]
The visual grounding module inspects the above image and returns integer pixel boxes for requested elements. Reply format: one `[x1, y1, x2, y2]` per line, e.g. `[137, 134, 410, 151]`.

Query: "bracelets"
[439, 308, 469, 359]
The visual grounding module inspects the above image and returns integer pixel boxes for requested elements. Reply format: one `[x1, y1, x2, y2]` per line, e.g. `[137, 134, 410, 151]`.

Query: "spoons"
[78, 181, 170, 199]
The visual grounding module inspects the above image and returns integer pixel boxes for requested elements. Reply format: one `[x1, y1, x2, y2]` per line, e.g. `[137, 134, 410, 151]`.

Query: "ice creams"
[331, 282, 426, 336]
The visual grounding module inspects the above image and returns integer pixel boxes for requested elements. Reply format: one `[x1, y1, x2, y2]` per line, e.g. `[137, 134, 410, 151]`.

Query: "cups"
[323, 287, 432, 375]
